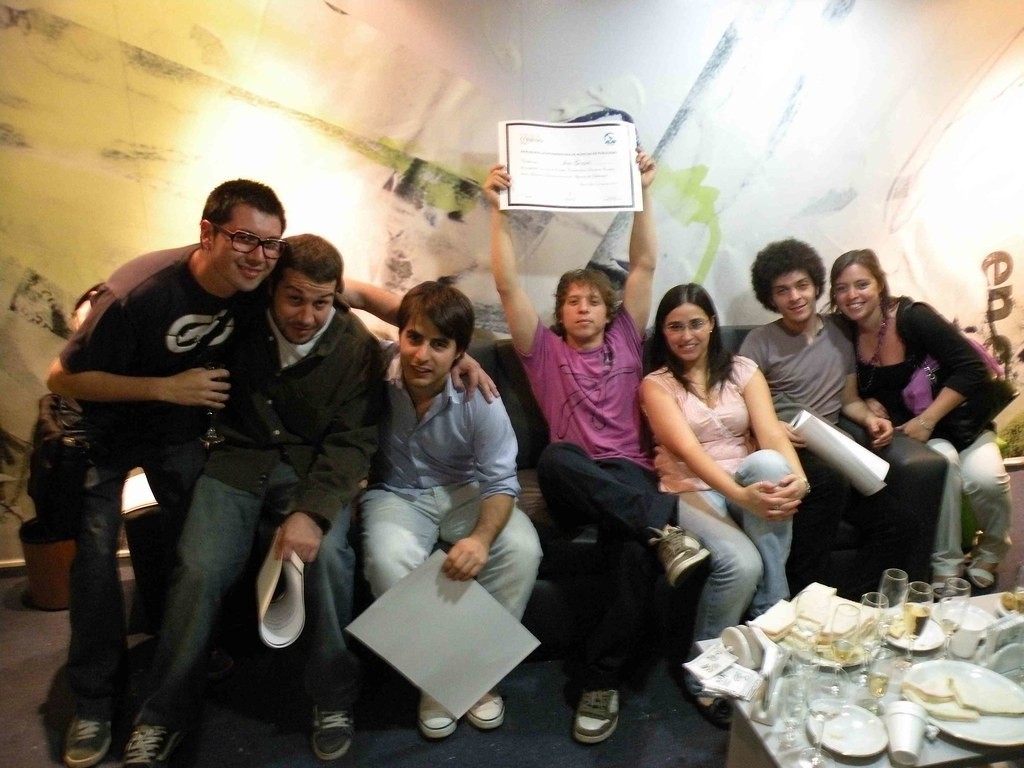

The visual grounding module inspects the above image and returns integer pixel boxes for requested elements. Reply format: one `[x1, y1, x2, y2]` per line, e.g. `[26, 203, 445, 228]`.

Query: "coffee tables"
[691, 591, 1024, 768]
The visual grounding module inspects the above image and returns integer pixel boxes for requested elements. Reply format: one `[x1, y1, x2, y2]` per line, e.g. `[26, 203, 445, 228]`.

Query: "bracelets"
[916, 415, 935, 432]
[802, 477, 812, 497]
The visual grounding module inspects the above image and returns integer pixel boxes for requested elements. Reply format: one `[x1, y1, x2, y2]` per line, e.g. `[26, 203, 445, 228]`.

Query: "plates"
[794, 635, 868, 668]
[931, 602, 997, 636]
[878, 612, 946, 653]
[806, 702, 889, 758]
[902, 659, 1024, 747]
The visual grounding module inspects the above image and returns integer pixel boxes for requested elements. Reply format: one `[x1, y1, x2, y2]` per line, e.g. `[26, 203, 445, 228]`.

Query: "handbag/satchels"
[903, 338, 1020, 446]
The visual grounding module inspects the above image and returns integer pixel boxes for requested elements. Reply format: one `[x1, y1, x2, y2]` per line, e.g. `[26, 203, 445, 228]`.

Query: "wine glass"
[768, 567, 971, 768]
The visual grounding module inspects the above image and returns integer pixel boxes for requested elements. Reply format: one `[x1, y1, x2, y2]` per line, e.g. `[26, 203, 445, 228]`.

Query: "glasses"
[212, 222, 289, 260]
[664, 315, 712, 333]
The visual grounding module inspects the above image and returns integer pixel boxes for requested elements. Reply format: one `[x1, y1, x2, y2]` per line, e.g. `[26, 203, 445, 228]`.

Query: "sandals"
[931, 574, 955, 598]
[966, 547, 998, 588]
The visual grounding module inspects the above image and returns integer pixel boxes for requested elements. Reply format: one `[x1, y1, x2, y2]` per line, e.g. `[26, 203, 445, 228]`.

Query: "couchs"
[124, 325, 948, 690]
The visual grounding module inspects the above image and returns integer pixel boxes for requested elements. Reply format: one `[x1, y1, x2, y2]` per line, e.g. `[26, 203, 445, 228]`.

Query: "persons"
[635, 281, 815, 736]
[828, 247, 1014, 600]
[45, 175, 546, 768]
[478, 143, 712, 745]
[737, 239, 922, 603]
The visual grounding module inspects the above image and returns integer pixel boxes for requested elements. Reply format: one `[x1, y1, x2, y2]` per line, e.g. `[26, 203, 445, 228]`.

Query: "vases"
[17, 516, 77, 610]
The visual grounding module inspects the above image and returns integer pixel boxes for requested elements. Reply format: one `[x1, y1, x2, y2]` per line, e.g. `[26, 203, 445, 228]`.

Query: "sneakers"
[65, 716, 113, 767]
[467, 686, 505, 728]
[313, 700, 353, 760]
[421, 692, 456, 739]
[124, 724, 180, 768]
[648, 526, 712, 589]
[575, 689, 618, 742]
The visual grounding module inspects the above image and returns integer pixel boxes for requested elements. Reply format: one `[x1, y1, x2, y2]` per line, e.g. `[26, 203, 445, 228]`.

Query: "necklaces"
[849, 315, 889, 392]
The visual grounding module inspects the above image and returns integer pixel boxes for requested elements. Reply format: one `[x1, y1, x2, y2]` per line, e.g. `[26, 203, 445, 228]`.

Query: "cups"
[1014, 560, 1024, 616]
[947, 613, 986, 658]
[887, 699, 928, 765]
